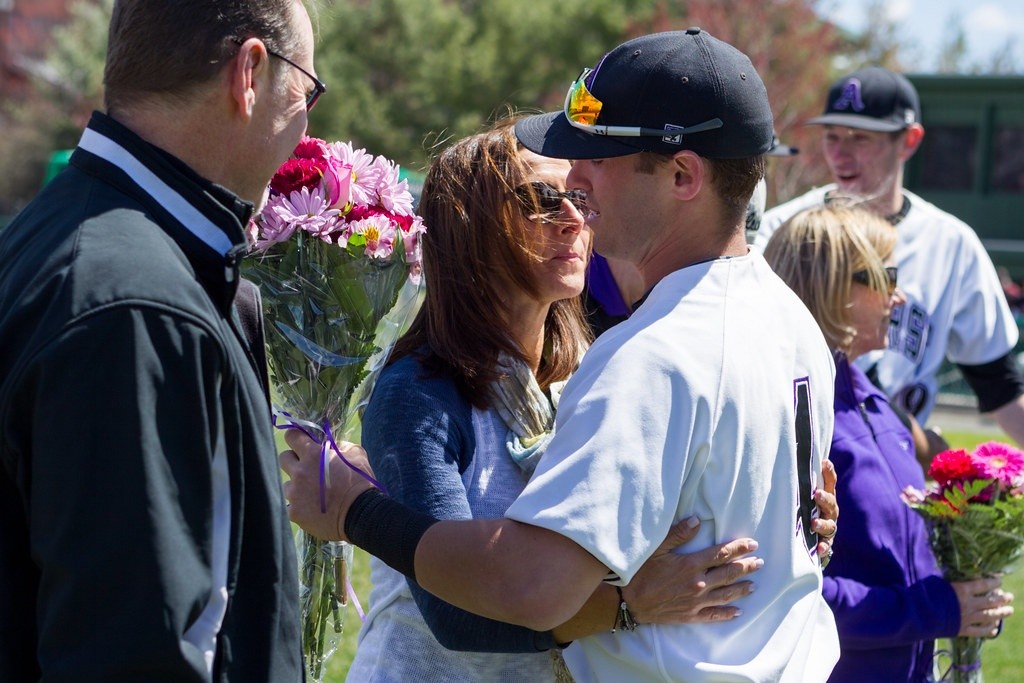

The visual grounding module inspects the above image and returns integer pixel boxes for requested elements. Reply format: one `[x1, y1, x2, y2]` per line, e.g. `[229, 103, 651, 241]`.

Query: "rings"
[824, 519, 837, 538]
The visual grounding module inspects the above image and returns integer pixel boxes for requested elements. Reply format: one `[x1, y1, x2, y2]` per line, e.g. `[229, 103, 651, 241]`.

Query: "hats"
[513, 28, 755, 161]
[804, 65, 924, 139]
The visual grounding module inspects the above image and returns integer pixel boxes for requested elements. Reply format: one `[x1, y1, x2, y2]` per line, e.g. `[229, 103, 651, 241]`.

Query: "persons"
[1, 1, 326, 683]
[279, 26, 1024, 683]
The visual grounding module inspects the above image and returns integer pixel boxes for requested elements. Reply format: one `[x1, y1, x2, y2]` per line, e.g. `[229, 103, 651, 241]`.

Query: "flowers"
[238, 132, 427, 680]
[900, 441, 1024, 683]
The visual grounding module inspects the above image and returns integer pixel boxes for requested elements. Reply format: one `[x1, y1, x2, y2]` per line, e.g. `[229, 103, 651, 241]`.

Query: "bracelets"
[611, 586, 640, 633]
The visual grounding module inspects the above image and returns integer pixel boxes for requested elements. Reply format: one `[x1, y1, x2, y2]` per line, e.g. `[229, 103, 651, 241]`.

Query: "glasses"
[502, 180, 592, 225]
[561, 64, 724, 137]
[261, 49, 327, 112]
[849, 265, 902, 290]
